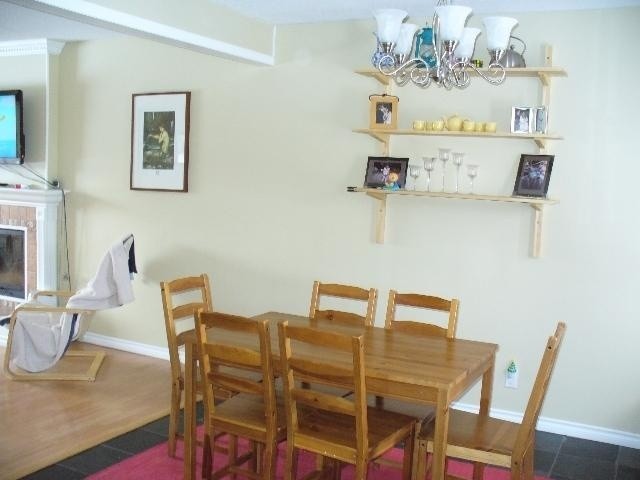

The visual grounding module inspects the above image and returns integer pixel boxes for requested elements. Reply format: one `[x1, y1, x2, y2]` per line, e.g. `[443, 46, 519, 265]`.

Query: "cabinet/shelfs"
[346, 43, 567, 259]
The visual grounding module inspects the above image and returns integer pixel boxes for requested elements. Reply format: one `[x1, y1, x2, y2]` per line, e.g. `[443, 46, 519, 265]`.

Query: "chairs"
[3, 232, 136, 382]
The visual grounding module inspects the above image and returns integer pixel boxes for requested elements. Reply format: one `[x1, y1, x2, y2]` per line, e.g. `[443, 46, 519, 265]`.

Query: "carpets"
[87, 423, 550, 480]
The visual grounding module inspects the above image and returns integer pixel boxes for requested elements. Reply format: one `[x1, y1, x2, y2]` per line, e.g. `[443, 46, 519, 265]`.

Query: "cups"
[412, 113, 497, 133]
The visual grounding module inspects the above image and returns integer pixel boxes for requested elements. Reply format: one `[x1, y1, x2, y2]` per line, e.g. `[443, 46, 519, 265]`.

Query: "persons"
[148, 125, 169, 155]
[520, 112, 528, 131]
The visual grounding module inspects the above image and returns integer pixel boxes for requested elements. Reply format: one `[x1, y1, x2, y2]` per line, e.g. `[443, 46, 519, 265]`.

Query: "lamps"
[371, 0, 520, 90]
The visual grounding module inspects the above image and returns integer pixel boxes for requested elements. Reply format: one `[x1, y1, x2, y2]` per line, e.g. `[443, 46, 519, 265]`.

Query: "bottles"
[415, 27, 437, 68]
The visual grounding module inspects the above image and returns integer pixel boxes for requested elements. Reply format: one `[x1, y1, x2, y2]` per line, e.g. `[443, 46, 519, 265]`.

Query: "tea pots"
[487, 36, 526, 68]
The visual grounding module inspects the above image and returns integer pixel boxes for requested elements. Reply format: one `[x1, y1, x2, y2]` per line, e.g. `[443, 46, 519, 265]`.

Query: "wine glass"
[409, 148, 479, 193]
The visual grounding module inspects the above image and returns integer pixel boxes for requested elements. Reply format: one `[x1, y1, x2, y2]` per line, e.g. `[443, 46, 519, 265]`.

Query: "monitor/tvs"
[0, 89, 25, 165]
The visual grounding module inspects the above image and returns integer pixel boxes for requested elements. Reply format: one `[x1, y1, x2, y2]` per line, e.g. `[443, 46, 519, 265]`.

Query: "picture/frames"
[363, 94, 555, 198]
[129, 91, 191, 193]
